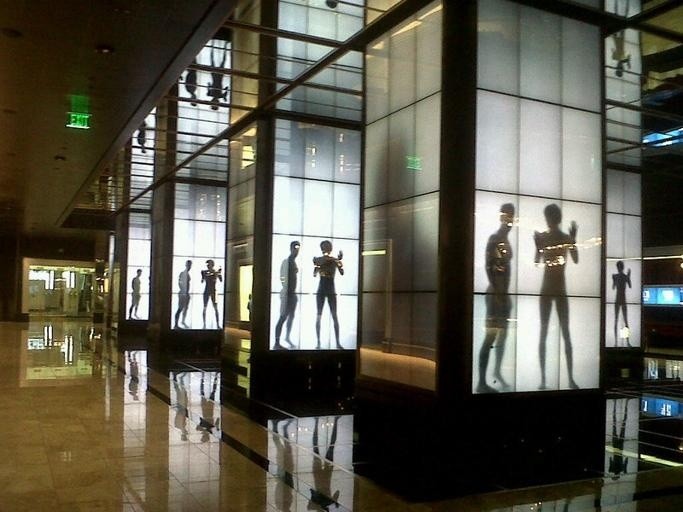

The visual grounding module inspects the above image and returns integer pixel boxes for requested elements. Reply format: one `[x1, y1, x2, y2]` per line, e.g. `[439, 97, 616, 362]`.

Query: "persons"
[313, 241, 344, 350]
[476, 204, 517, 393]
[127, 351, 139, 400]
[138, 122, 147, 153]
[612, 30, 632, 77]
[196, 373, 220, 442]
[201, 260, 222, 330]
[609, 399, 629, 481]
[172, 372, 189, 441]
[186, 59, 198, 106]
[271, 420, 294, 512]
[307, 416, 340, 512]
[533, 204, 580, 389]
[612, 262, 632, 346]
[274, 240, 300, 349]
[207, 39, 229, 110]
[129, 269, 142, 320]
[174, 260, 192, 329]
